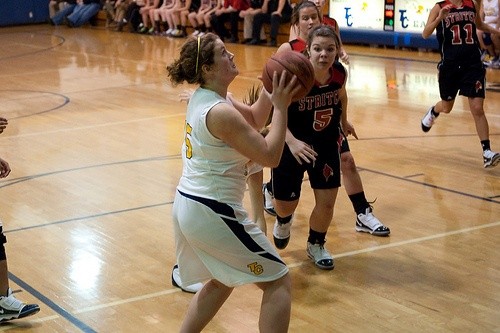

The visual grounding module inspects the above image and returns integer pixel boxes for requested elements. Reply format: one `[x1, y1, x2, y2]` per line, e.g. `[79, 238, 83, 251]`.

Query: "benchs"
[98, 10, 292, 46]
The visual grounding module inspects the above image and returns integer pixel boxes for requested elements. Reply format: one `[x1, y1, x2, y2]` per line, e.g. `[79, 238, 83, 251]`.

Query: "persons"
[421, 0, 500, 169]
[0, 116, 40, 322]
[263, 0, 390, 269]
[164, 30, 301, 333]
[49, 0, 293, 47]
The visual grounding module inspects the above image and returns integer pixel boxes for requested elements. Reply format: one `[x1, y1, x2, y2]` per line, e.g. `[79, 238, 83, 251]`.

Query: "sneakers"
[273, 215, 294, 250]
[171, 265, 203, 293]
[422, 105, 439, 132]
[137, 24, 205, 39]
[482, 148, 500, 169]
[263, 183, 276, 215]
[105, 18, 126, 32]
[219, 31, 277, 47]
[354, 207, 392, 236]
[0, 288, 40, 322]
[306, 241, 335, 270]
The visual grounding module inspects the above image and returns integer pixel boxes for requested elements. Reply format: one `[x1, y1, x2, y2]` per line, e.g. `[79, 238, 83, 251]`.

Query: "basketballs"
[261, 48, 316, 103]
